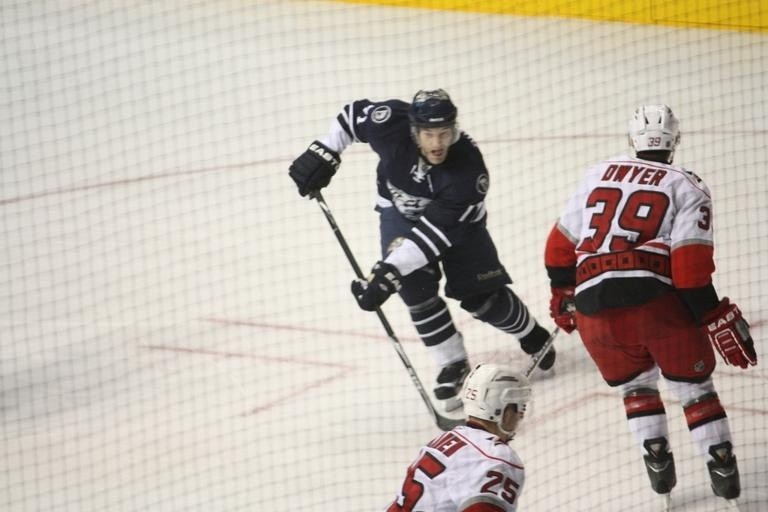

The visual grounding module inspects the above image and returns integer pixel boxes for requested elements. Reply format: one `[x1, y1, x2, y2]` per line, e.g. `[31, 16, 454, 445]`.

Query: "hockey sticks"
[312, 187, 464, 431]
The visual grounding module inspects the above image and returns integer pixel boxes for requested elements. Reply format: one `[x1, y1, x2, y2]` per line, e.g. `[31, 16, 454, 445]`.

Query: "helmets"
[462, 360, 531, 420]
[407, 88, 458, 126]
[626, 102, 681, 153]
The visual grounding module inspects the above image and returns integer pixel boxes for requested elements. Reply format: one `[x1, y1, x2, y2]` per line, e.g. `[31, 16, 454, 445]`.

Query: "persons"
[383, 362, 532, 512]
[544, 105, 757, 499]
[289, 88, 556, 401]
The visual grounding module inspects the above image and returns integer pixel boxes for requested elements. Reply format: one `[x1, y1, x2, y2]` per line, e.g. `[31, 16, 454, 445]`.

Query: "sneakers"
[703, 445, 743, 497]
[515, 324, 561, 369]
[646, 443, 677, 496]
[430, 358, 470, 405]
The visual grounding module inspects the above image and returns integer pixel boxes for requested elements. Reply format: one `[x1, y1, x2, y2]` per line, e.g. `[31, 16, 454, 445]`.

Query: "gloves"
[549, 286, 576, 332]
[289, 141, 342, 199]
[705, 296, 755, 367]
[346, 259, 402, 314]
